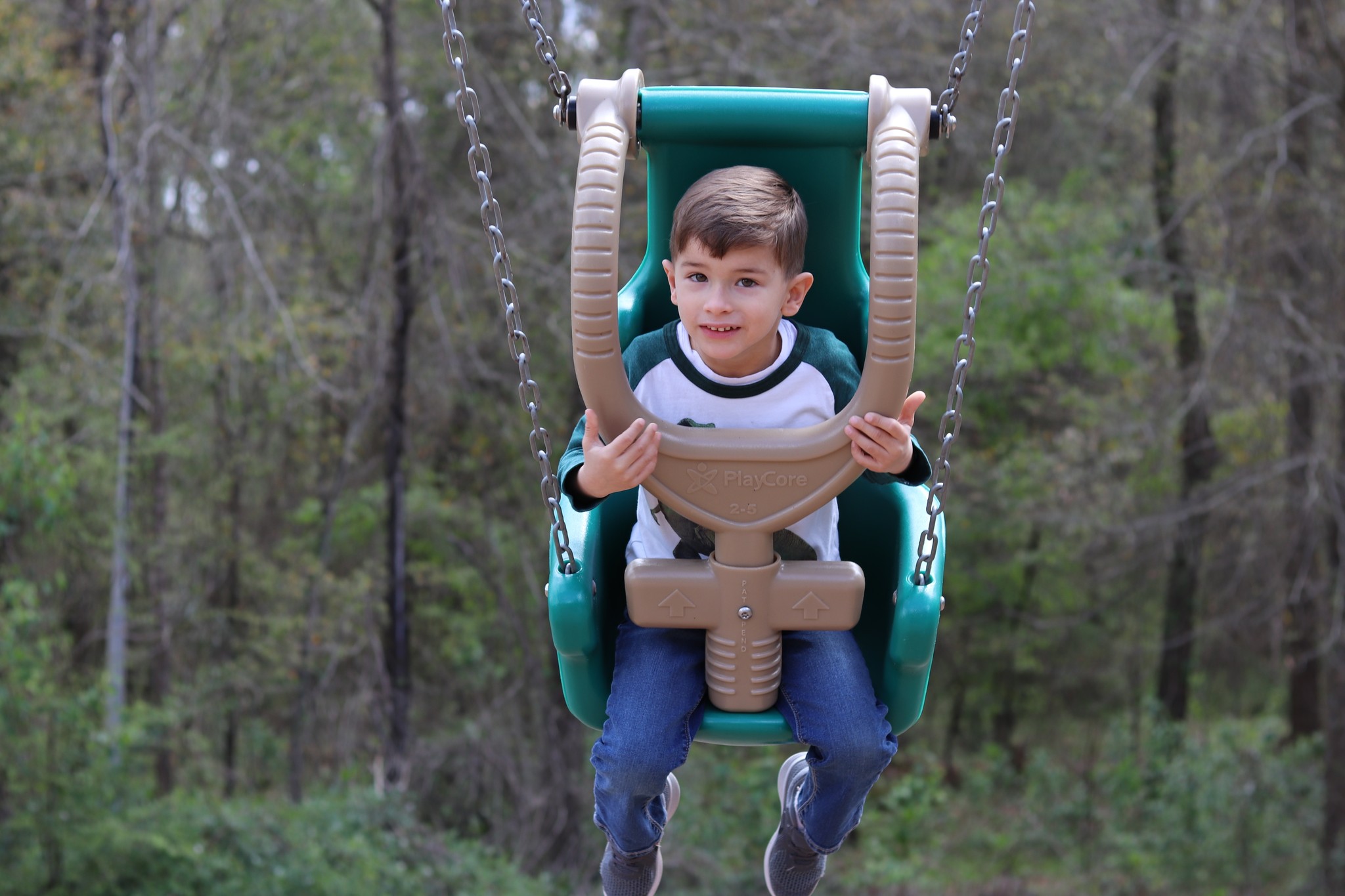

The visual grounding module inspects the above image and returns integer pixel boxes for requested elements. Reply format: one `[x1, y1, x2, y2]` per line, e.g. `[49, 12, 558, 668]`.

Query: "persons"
[554, 166, 931, 896]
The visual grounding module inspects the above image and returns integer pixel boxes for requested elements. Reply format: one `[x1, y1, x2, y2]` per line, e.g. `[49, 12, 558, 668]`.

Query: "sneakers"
[600, 771, 680, 896]
[763, 750, 828, 896]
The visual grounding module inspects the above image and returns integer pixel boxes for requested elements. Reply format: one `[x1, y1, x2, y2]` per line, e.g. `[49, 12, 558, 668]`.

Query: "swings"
[429, 0, 1041, 756]
